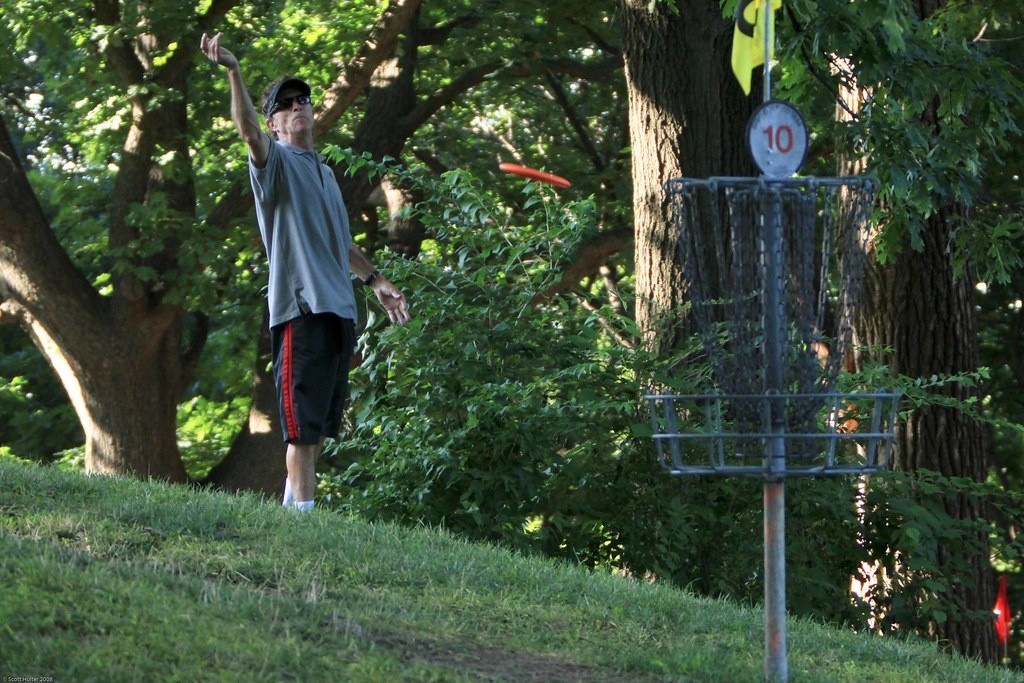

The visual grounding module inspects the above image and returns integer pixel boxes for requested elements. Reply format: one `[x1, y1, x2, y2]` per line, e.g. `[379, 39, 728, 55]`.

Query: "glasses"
[270, 94, 310, 115]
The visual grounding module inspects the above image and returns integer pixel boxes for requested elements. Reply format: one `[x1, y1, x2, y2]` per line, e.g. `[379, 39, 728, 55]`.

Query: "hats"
[262, 78, 311, 116]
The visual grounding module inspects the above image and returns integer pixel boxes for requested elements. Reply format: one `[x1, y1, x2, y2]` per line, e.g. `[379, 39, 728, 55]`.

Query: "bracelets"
[363, 269, 380, 287]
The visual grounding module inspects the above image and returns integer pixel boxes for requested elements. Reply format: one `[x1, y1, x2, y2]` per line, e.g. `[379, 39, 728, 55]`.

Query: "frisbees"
[499, 162, 575, 188]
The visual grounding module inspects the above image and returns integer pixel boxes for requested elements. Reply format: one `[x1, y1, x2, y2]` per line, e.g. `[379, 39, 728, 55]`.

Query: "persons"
[197, 29, 410, 511]
